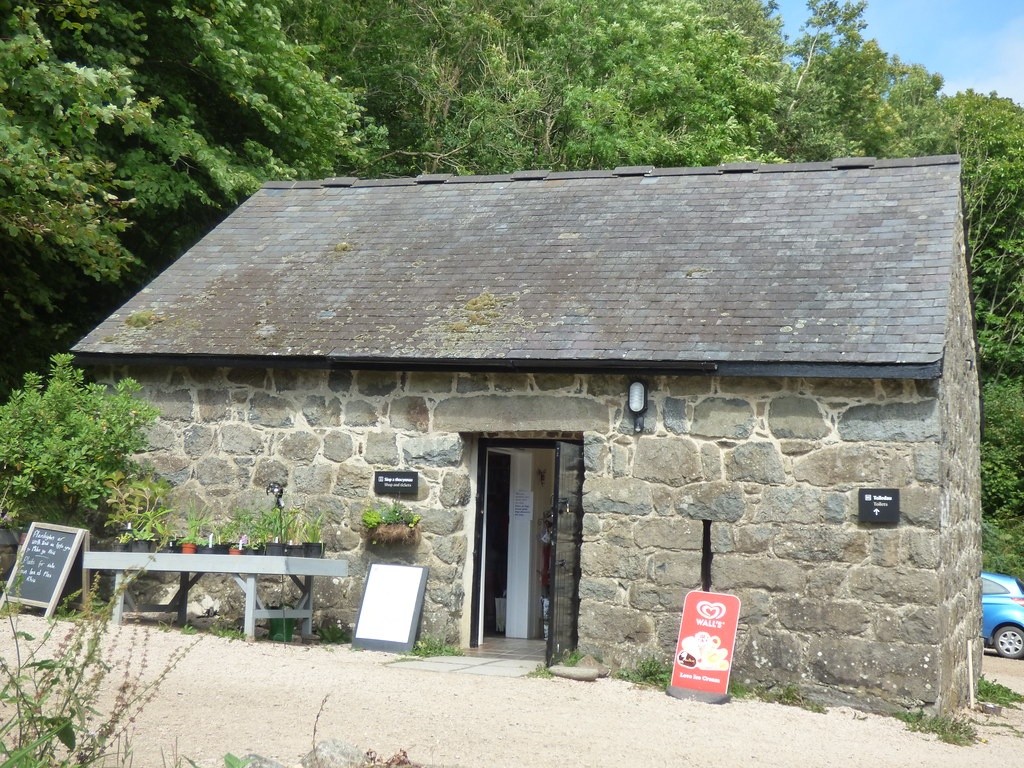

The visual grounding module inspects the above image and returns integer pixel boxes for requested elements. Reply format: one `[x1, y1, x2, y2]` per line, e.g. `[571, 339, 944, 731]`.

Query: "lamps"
[628, 377, 648, 433]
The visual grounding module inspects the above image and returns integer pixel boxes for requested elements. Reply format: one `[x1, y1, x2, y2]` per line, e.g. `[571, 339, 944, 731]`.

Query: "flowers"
[104, 472, 325, 548]
[360, 501, 420, 526]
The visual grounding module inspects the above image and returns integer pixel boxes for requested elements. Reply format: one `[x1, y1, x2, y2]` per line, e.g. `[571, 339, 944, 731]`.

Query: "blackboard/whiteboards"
[2, 521, 86, 609]
[352, 562, 429, 653]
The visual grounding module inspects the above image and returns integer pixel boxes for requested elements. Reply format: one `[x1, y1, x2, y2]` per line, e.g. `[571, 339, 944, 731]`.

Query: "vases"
[105, 539, 330, 559]
[369, 525, 420, 547]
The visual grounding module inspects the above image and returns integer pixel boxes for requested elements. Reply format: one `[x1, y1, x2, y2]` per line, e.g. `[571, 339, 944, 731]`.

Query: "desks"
[81, 551, 349, 647]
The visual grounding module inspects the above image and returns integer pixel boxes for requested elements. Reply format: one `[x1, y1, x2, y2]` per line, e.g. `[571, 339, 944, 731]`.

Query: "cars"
[980, 571, 1024, 660]
